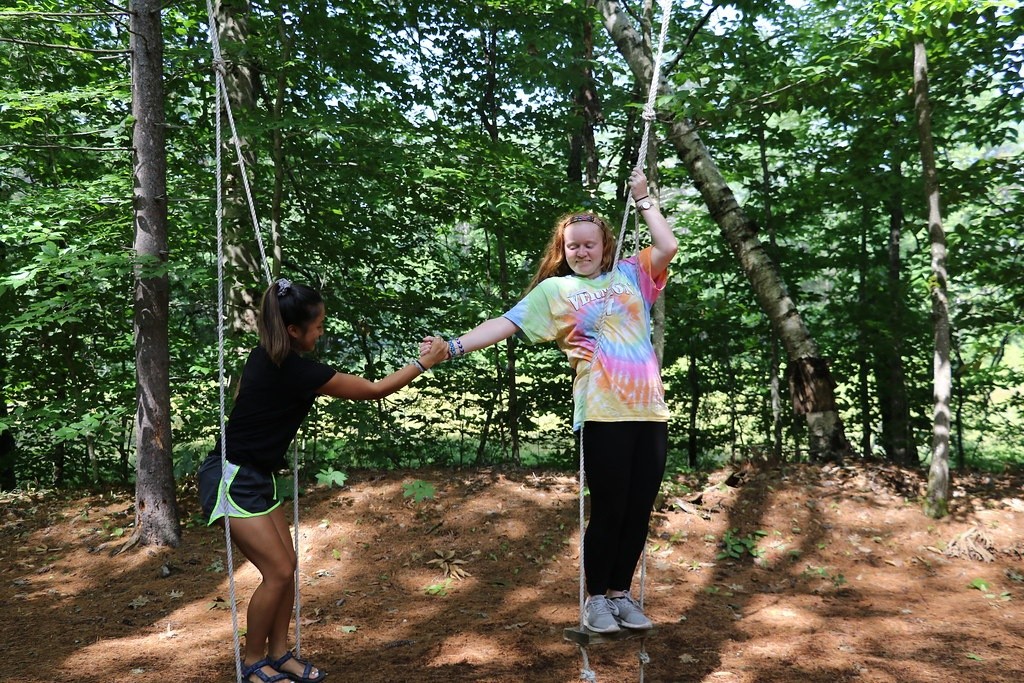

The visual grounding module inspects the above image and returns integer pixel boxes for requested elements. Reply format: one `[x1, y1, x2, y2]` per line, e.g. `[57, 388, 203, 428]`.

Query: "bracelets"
[636, 194, 649, 202]
[413, 362, 424, 373]
[417, 360, 427, 371]
[455, 338, 464, 355]
[448, 340, 456, 357]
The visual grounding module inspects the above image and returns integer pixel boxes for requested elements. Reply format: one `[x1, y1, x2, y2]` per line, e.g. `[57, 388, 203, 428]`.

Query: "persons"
[197, 279, 450, 683]
[420, 167, 678, 632]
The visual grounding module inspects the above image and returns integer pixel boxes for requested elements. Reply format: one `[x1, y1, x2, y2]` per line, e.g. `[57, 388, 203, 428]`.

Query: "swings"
[565, 0, 674, 645]
[203, 5, 300, 682]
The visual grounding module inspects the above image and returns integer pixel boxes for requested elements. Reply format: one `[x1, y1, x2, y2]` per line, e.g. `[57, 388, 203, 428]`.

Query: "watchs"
[638, 202, 655, 212]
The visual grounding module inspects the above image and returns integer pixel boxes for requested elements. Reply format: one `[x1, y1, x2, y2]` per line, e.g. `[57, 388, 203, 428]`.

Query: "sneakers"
[583, 590, 653, 632]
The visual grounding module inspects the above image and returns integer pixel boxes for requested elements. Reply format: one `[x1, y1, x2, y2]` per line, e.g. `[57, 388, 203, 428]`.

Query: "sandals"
[241, 651, 324, 683]
